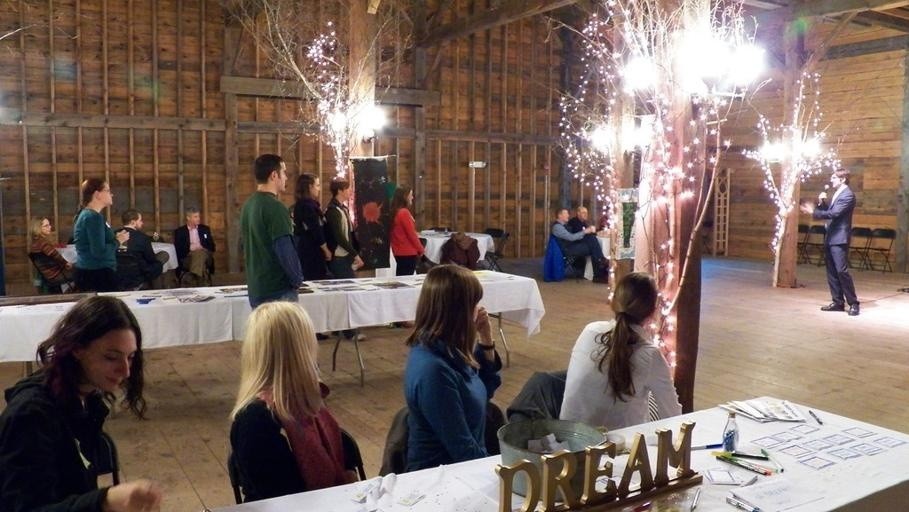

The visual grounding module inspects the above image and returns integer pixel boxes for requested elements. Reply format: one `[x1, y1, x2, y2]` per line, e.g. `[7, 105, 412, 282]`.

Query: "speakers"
[352, 154, 392, 270]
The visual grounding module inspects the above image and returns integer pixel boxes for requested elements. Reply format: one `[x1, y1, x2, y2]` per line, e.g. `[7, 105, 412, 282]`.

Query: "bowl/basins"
[495, 419, 606, 503]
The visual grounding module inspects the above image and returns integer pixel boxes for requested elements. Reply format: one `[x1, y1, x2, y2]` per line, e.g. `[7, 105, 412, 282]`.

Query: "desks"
[211, 394, 909, 511]
[417, 231, 496, 265]
[58, 241, 179, 290]
[0, 268, 545, 385]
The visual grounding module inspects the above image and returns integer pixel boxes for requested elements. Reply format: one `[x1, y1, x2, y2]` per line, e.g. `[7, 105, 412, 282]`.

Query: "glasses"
[98, 189, 113, 193]
[39, 224, 50, 228]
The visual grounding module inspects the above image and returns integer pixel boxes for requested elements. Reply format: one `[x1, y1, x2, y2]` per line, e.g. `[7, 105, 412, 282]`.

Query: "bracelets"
[479, 340, 498, 352]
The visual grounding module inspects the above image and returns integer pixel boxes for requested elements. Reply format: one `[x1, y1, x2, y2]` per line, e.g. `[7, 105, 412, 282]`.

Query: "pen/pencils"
[711, 449, 784, 475]
[690, 444, 723, 450]
[726, 491, 762, 512]
[744, 475, 757, 486]
[809, 410, 823, 426]
[691, 487, 701, 512]
[629, 500, 652, 512]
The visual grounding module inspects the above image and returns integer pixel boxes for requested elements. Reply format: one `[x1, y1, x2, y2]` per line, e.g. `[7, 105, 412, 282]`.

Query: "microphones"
[821, 183, 830, 201]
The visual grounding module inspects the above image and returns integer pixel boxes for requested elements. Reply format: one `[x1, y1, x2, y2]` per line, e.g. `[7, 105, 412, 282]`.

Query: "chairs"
[24, 248, 76, 294]
[227, 427, 367, 505]
[549, 235, 581, 285]
[179, 268, 213, 287]
[509, 370, 567, 449]
[484, 228, 510, 273]
[797, 223, 896, 274]
[379, 401, 506, 477]
[429, 227, 452, 231]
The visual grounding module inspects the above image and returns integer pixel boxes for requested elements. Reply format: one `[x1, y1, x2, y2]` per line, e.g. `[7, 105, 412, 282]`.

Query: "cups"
[605, 434, 625, 451]
[118, 241, 128, 254]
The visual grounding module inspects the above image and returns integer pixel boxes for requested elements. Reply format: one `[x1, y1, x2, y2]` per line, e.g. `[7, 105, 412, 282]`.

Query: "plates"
[135, 298, 154, 305]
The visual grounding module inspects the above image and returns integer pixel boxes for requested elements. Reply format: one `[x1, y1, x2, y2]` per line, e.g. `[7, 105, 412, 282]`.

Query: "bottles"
[724, 411, 739, 453]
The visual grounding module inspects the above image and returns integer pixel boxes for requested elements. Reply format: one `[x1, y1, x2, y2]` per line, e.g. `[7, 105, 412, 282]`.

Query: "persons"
[799, 169, 861, 315]
[323, 177, 368, 342]
[0, 295, 164, 512]
[237, 153, 304, 309]
[560, 272, 684, 432]
[403, 265, 502, 475]
[287, 172, 332, 340]
[549, 202, 616, 282]
[27, 178, 215, 292]
[384, 184, 422, 328]
[227, 300, 359, 504]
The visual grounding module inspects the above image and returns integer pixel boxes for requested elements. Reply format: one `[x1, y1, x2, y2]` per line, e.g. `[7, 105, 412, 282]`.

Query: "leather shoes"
[849, 303, 860, 316]
[821, 301, 843, 310]
